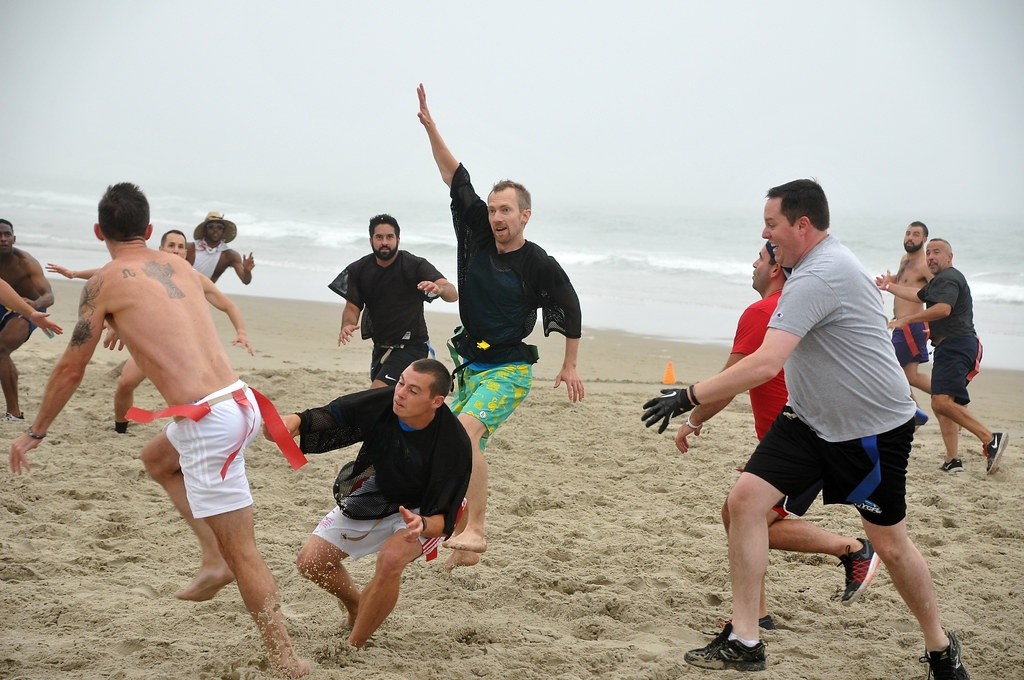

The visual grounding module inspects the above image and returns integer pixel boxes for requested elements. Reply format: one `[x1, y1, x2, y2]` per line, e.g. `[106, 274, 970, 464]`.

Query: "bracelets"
[885, 281, 893, 291]
[417, 513, 427, 532]
[28, 426, 46, 439]
[689, 384, 700, 406]
[684, 414, 701, 430]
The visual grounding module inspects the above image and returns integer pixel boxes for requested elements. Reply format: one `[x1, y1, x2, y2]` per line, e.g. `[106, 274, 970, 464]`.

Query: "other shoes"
[0, 412, 24, 421]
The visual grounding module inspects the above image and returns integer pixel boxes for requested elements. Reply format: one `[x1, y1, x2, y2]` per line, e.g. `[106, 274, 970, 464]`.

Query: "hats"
[766, 239, 793, 280]
[194, 211, 237, 244]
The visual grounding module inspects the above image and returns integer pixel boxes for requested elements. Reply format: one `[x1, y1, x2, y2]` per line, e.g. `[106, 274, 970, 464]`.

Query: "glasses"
[207, 223, 225, 231]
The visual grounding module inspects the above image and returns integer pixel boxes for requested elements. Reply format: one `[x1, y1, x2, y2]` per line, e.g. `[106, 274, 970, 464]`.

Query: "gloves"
[640, 385, 700, 434]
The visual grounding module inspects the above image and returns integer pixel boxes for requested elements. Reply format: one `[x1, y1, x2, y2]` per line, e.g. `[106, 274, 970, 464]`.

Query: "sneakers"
[940, 458, 964, 473]
[837, 538, 881, 607]
[919, 631, 970, 680]
[684, 623, 766, 671]
[982, 432, 1008, 475]
[759, 614, 773, 630]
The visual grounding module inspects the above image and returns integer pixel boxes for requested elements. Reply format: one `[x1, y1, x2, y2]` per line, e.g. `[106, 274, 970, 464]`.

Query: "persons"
[0, 278, 64, 338]
[675, 240, 881, 605]
[0, 220, 54, 421]
[45, 229, 254, 435]
[326, 214, 459, 391]
[107, 212, 254, 378]
[877, 237, 1009, 476]
[415, 81, 586, 573]
[874, 221, 962, 432]
[10, 182, 308, 679]
[640, 180, 968, 680]
[260, 359, 472, 649]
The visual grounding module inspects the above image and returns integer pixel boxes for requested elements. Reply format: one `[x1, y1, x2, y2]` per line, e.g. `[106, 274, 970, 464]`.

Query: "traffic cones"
[662, 363, 677, 386]
